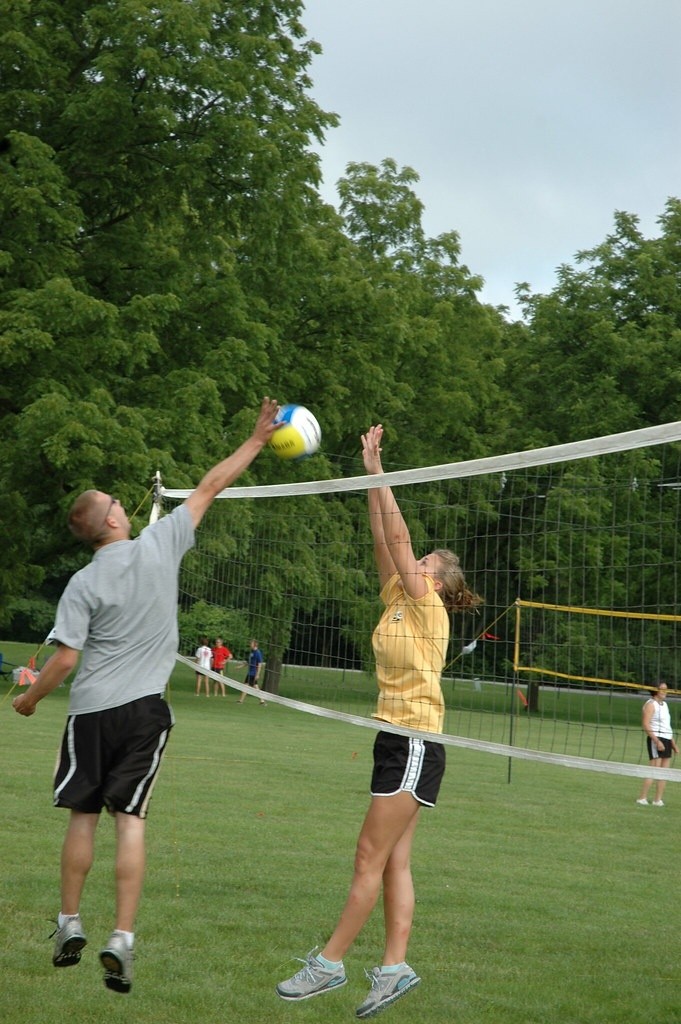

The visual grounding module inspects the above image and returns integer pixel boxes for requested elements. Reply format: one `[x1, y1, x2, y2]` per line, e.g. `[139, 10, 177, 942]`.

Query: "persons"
[195, 639, 213, 697]
[277, 424, 481, 1019]
[637, 678, 679, 805]
[234, 639, 265, 704]
[211, 638, 233, 697]
[11, 397, 287, 994]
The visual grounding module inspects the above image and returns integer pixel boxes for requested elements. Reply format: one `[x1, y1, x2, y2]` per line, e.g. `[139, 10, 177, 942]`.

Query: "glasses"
[102, 500, 118, 526]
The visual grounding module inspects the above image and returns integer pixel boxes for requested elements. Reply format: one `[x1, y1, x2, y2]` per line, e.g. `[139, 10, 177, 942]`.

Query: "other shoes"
[259, 701, 267, 706]
[652, 800, 664, 806]
[236, 700, 243, 704]
[636, 799, 648, 805]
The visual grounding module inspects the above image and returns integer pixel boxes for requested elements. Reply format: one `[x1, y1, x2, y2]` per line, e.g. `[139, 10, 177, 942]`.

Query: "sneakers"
[356, 966, 422, 1018]
[45, 917, 86, 966]
[274, 946, 347, 1001]
[98, 934, 133, 993]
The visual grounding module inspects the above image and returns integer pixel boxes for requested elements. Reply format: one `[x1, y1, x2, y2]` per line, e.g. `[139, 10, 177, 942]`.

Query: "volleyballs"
[267, 403, 322, 462]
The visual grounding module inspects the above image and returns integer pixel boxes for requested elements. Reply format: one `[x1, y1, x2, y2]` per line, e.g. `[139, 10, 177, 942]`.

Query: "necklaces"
[653, 698, 663, 705]
